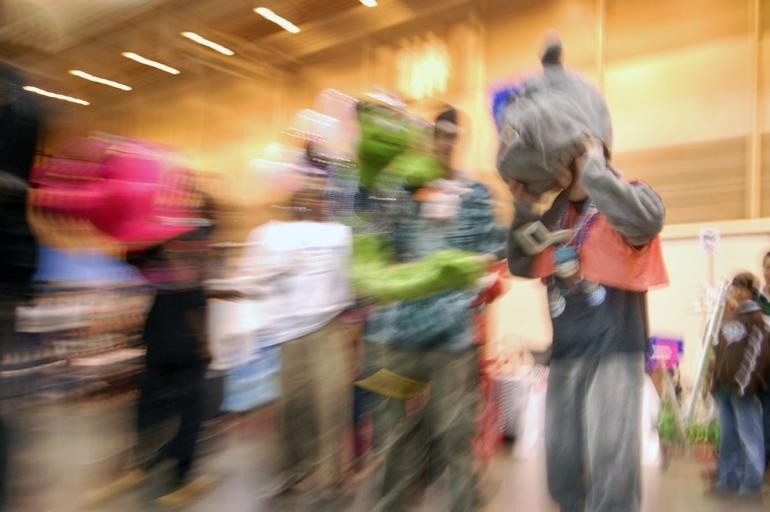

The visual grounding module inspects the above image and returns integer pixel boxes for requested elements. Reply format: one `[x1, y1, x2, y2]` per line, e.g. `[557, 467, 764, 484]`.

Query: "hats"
[732, 271, 759, 299]
